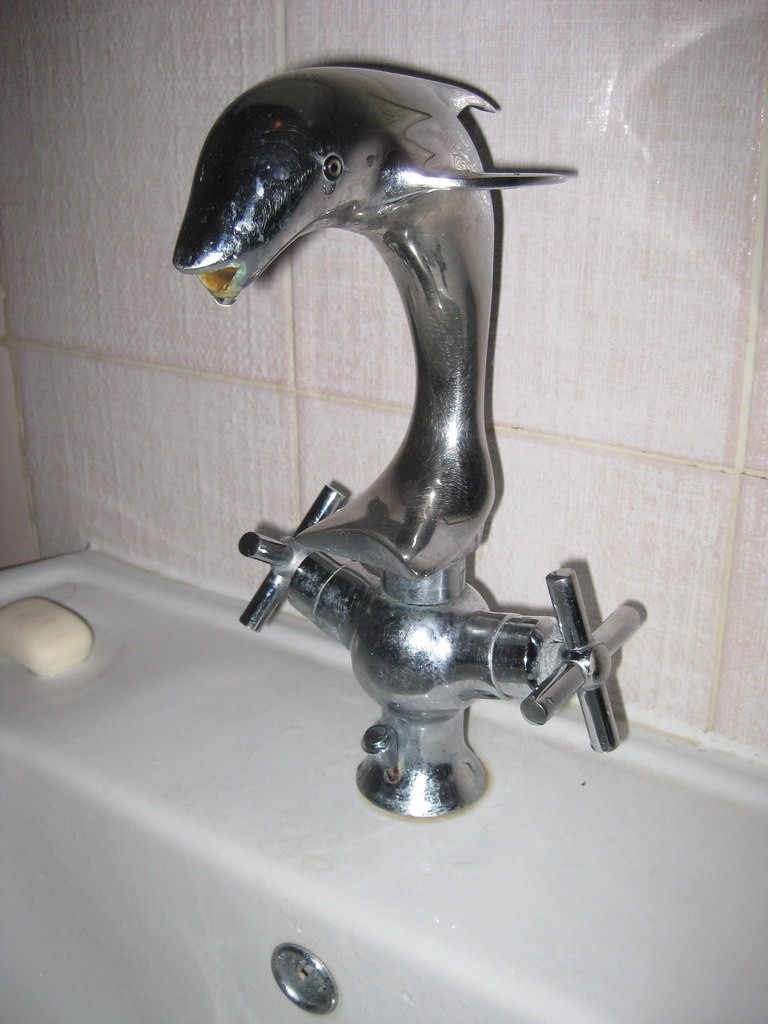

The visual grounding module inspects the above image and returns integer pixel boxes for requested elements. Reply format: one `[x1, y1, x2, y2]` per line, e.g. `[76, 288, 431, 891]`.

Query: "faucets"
[168, 63, 647, 821]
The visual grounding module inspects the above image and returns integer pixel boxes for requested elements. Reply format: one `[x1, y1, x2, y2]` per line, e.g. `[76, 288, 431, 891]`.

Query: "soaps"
[0, 595, 92, 676]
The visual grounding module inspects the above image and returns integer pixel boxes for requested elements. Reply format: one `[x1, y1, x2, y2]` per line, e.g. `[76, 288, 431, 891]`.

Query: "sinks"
[0, 555, 768, 1016]
